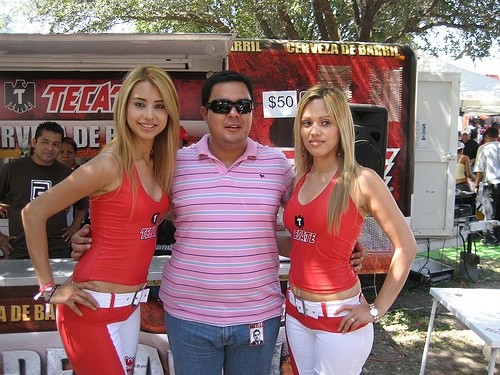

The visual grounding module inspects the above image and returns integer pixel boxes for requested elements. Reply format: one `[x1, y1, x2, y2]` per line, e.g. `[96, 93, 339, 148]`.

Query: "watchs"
[369, 303, 378, 321]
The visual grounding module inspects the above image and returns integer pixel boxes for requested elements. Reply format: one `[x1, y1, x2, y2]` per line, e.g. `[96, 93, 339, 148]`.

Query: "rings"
[351, 318, 355, 322]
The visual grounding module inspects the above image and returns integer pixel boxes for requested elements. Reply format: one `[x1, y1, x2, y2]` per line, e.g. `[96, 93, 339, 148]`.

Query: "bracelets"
[47, 286, 58, 302]
[34, 281, 54, 301]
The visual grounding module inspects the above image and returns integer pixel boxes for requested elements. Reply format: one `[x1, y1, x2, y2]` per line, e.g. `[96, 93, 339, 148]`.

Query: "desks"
[0, 253, 291, 286]
[420, 288, 500, 375]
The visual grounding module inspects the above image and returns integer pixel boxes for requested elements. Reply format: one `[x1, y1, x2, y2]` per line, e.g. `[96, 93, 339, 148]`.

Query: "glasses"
[205, 97, 255, 115]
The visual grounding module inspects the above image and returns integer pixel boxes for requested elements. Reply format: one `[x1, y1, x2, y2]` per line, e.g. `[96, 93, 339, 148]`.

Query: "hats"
[458, 141, 465, 150]
[492, 122, 500, 127]
[460, 125, 472, 135]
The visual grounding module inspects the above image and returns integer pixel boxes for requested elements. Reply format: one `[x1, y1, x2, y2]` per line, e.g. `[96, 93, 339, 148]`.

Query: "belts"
[82, 283, 150, 309]
[287, 282, 361, 320]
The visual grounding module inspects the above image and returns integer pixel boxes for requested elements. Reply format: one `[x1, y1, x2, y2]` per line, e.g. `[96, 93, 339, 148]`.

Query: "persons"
[456, 141, 478, 238]
[473, 128, 500, 246]
[457, 115, 500, 147]
[458, 127, 479, 175]
[21, 65, 179, 375]
[71, 70, 365, 375]
[0, 121, 88, 259]
[54, 137, 89, 225]
[282, 83, 416, 375]
